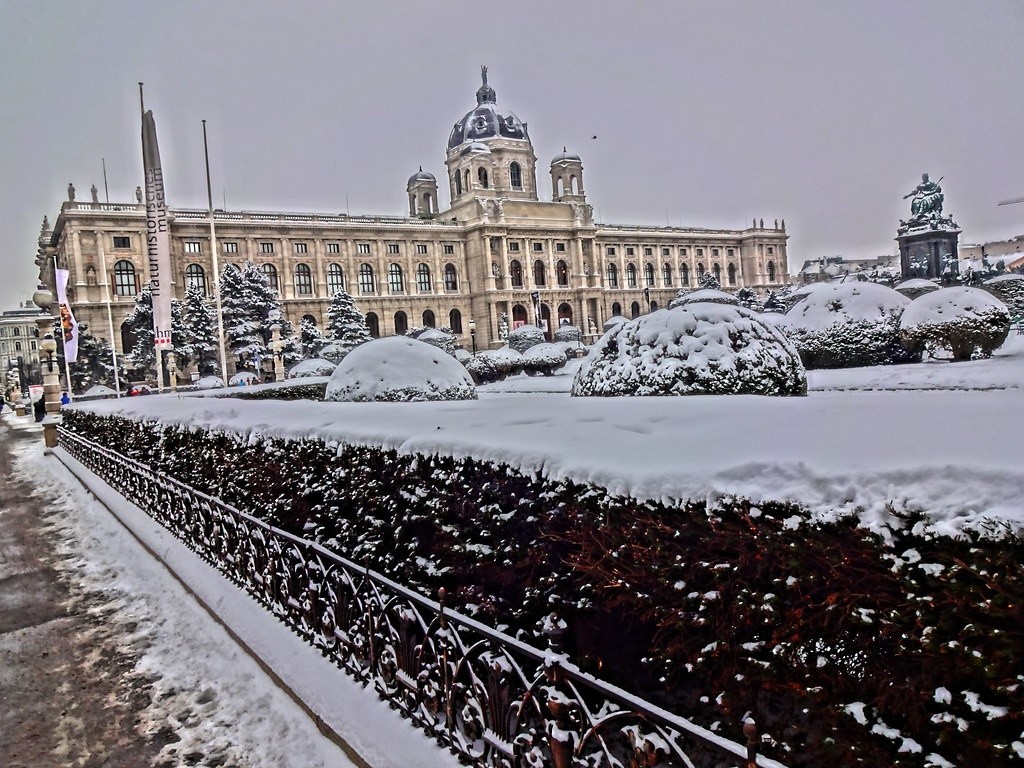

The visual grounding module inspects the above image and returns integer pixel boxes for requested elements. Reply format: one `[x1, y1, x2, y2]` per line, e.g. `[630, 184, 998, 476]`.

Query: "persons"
[68, 183, 75, 202]
[91, 185, 98, 203]
[589, 205, 593, 218]
[125, 381, 158, 397]
[584, 264, 590, 274]
[497, 201, 503, 215]
[60, 393, 69, 404]
[136, 186, 142, 203]
[902, 173, 944, 220]
[856, 254, 1005, 287]
[43, 215, 49, 230]
[87, 268, 95, 285]
[947, 214, 961, 230]
[753, 218, 785, 229]
[897, 219, 909, 234]
[0, 398, 5, 413]
[492, 264, 500, 277]
[238, 373, 273, 386]
[575, 204, 581, 217]
[482, 200, 487, 213]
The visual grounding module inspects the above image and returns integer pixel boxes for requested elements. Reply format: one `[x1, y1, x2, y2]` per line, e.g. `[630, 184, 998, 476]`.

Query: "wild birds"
[592, 136, 597, 139]
